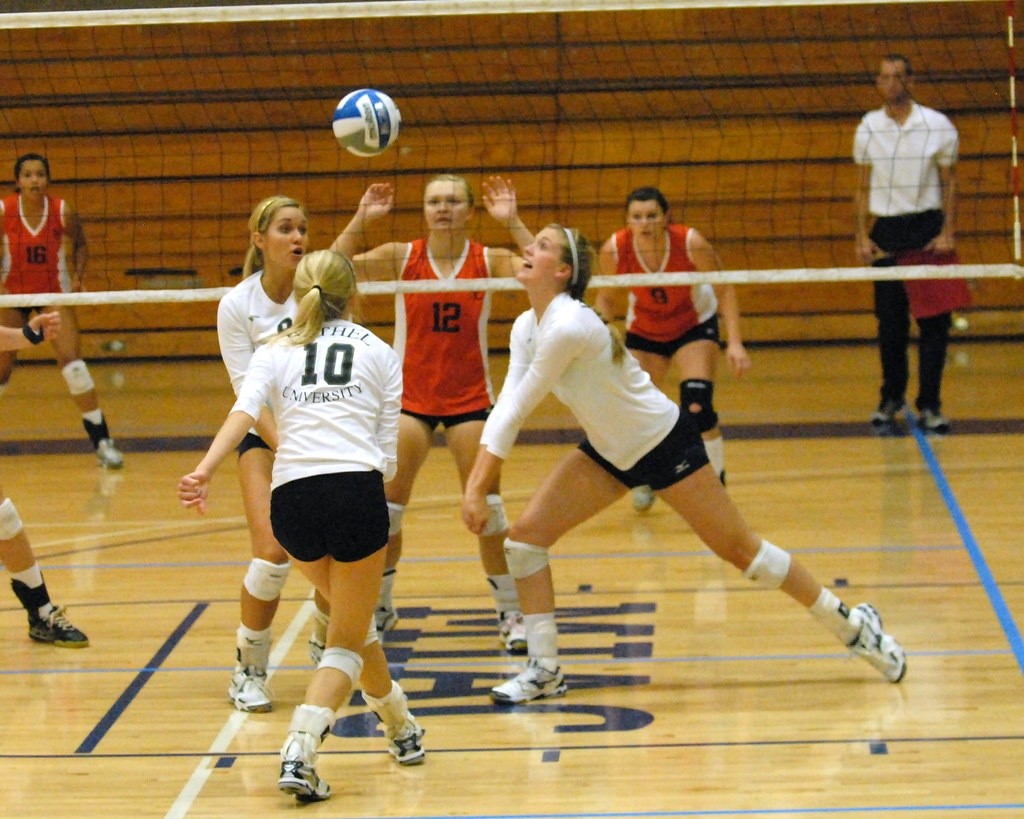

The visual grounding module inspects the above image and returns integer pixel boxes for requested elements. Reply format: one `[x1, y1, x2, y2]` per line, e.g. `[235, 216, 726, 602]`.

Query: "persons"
[596, 189, 749, 488]
[0, 311, 88, 647]
[852, 53, 960, 429]
[0, 154, 125, 469]
[178, 250, 426, 800]
[463, 224, 906, 701]
[330, 172, 534, 655]
[213, 198, 330, 712]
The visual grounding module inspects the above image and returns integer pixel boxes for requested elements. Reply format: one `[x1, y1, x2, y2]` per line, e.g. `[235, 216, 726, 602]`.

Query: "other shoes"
[872, 396, 905, 425]
[632, 485, 655, 511]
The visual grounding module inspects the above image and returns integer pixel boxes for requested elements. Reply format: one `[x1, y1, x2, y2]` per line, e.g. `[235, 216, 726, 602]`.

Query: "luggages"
[920, 408, 951, 432]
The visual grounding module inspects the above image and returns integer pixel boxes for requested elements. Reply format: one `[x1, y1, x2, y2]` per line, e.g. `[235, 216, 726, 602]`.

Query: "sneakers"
[278, 755, 331, 801]
[96, 438, 123, 468]
[490, 658, 568, 704]
[227, 664, 272, 712]
[375, 607, 397, 643]
[27, 604, 88, 648]
[386, 725, 426, 765]
[310, 632, 325, 666]
[845, 603, 907, 683]
[498, 611, 528, 652]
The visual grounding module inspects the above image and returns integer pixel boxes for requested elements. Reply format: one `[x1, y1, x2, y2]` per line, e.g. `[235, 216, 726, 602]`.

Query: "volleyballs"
[331, 88, 401, 158]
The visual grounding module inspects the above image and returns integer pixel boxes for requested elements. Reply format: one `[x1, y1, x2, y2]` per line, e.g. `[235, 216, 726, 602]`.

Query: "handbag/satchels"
[899, 251, 974, 318]
